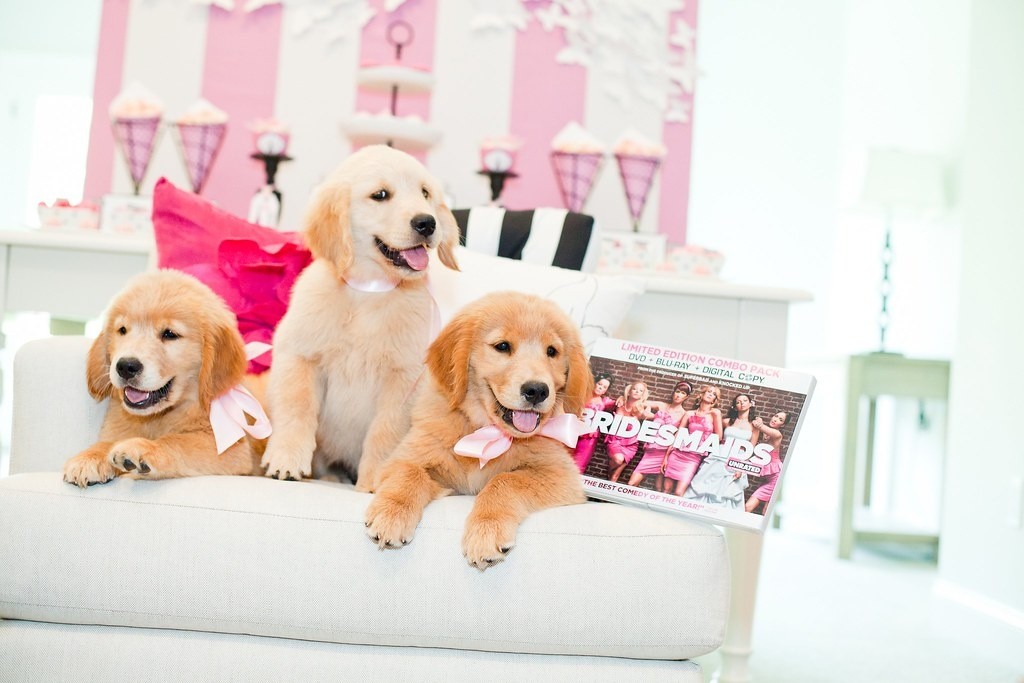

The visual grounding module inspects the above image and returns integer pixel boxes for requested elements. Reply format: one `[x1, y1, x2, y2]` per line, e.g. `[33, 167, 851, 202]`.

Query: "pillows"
[153, 174, 315, 376]
[429, 248, 647, 358]
[450, 205, 595, 273]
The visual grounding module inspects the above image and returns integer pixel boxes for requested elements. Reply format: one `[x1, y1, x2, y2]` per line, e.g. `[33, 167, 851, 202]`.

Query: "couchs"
[0, 336, 731, 683]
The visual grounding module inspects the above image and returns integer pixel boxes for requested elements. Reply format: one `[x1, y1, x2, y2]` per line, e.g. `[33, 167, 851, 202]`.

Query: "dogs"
[60, 268, 272, 487]
[254, 142, 464, 495]
[366, 289, 593, 569]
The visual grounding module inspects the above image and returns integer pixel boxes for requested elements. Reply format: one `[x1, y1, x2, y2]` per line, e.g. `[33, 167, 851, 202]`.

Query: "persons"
[745, 411, 792, 513]
[660, 386, 723, 497]
[615, 381, 693, 492]
[605, 382, 655, 482]
[565, 373, 616, 475]
[683, 394, 760, 512]
